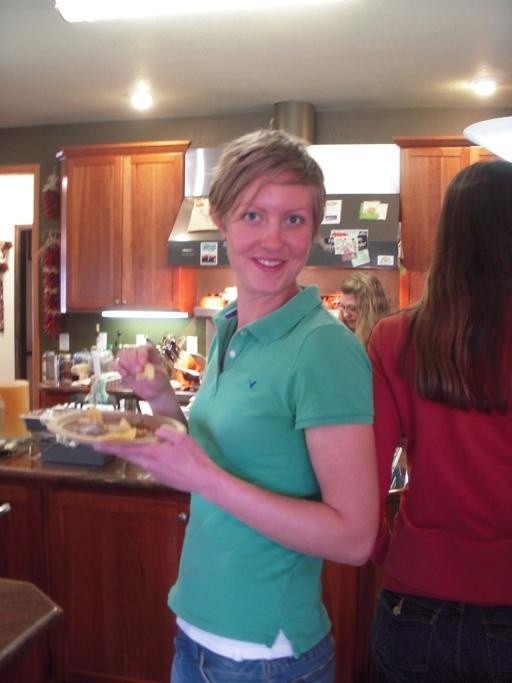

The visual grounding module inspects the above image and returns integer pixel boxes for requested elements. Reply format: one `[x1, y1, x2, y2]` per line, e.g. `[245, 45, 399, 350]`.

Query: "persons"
[86, 124, 385, 680]
[364, 157, 511, 681]
[334, 267, 394, 350]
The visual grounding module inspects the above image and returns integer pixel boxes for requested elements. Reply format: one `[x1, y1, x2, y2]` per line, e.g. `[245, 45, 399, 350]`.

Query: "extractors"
[167, 98, 402, 271]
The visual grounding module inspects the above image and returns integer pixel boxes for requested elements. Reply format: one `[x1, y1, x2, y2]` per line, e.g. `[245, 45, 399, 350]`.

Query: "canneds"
[41, 351, 58, 384]
[57, 353, 73, 386]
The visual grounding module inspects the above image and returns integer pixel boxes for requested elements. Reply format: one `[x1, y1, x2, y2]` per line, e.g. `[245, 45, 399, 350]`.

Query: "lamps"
[460, 115, 512, 163]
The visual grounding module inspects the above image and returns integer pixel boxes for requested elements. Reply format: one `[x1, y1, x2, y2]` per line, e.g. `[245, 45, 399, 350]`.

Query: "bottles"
[42, 350, 72, 386]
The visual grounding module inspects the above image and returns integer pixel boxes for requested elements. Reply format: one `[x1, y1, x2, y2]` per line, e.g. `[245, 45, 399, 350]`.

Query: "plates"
[45, 409, 188, 447]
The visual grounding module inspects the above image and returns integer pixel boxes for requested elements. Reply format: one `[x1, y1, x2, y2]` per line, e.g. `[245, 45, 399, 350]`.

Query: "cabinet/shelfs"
[53, 141, 238, 322]
[320, 497, 399, 681]
[3, 429, 189, 680]
[392, 132, 498, 310]
